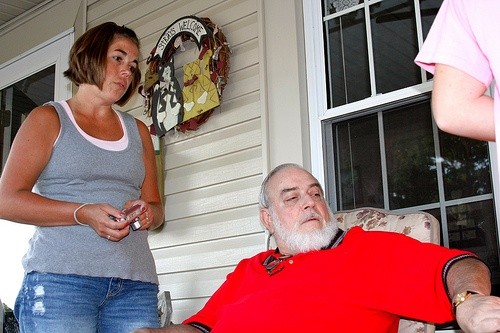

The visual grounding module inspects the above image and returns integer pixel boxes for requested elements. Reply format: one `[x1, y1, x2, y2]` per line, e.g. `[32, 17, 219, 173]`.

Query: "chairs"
[267, 207, 440, 333]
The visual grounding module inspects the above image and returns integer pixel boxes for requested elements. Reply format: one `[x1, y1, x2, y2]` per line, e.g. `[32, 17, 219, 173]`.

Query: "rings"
[108, 235, 111, 239]
[145, 212, 149, 219]
[147, 222, 151, 226]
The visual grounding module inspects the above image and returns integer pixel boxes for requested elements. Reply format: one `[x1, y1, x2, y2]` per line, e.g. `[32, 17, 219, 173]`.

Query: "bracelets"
[74, 202, 95, 226]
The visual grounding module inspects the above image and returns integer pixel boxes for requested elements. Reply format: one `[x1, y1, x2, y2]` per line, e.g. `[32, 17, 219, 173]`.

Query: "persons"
[0, 21, 169, 333]
[414, 0, 500, 177]
[133, 162, 500, 333]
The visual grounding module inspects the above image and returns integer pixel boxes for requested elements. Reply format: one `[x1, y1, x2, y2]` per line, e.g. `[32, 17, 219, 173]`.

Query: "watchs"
[451, 290, 479, 315]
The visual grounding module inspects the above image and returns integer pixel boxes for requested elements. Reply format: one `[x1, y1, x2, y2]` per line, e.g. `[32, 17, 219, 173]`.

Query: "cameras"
[112, 205, 146, 231]
